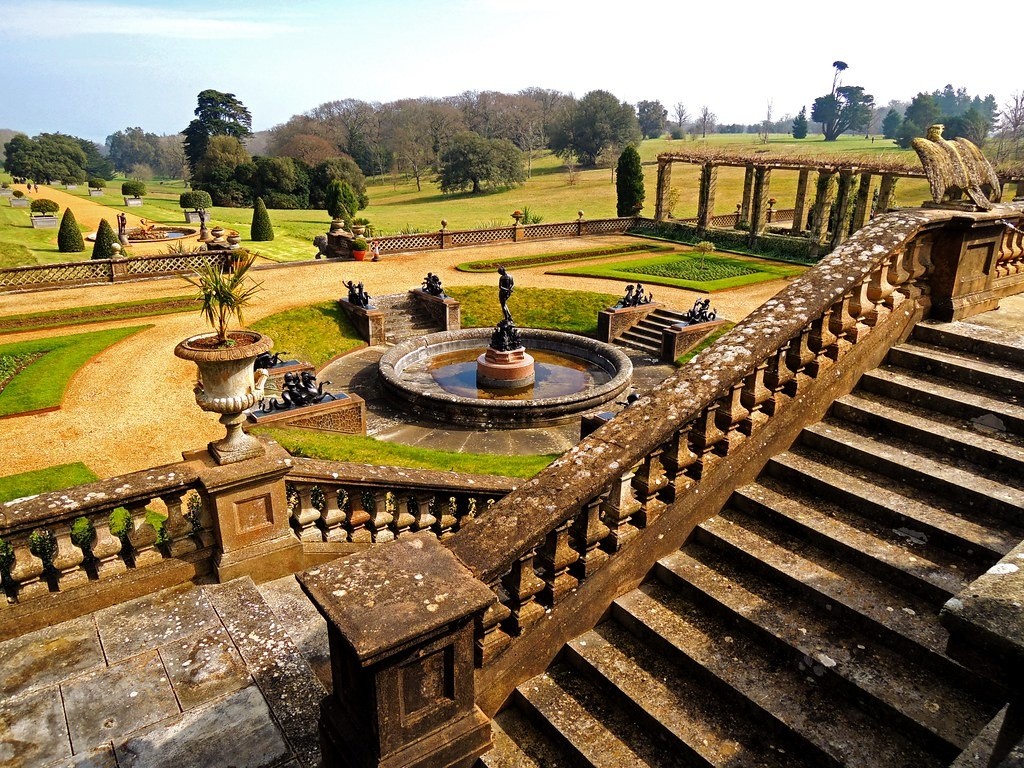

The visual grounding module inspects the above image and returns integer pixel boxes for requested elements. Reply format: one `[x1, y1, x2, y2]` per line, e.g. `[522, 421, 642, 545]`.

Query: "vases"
[332, 220, 345, 231]
[211, 229, 226, 241]
[509, 214, 525, 225]
[227, 235, 241, 247]
[766, 201, 776, 210]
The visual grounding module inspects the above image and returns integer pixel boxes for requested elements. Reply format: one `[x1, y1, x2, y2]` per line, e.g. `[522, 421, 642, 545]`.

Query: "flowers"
[769, 198, 776, 202]
[514, 210, 523, 215]
[215, 226, 222, 230]
[230, 231, 239, 236]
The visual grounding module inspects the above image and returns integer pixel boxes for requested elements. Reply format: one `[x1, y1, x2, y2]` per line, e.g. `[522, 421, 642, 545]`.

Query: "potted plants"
[351, 238, 368, 261]
[9, 191, 29, 208]
[30, 199, 60, 228]
[122, 180, 148, 206]
[0, 182, 14, 196]
[49, 174, 62, 185]
[65, 176, 78, 190]
[179, 189, 213, 223]
[88, 178, 107, 196]
[177, 250, 275, 465]
[351, 217, 369, 235]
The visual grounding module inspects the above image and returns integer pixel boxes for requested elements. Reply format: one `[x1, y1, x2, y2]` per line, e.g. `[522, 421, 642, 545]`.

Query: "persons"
[197, 209, 205, 229]
[284, 373, 306, 405]
[342, 281, 358, 298]
[34, 182, 38, 192]
[700, 299, 710, 321]
[632, 283, 642, 307]
[423, 272, 432, 283]
[14, 178, 17, 184]
[27, 183, 31, 193]
[21, 177, 24, 184]
[119, 213, 126, 233]
[497, 267, 515, 322]
